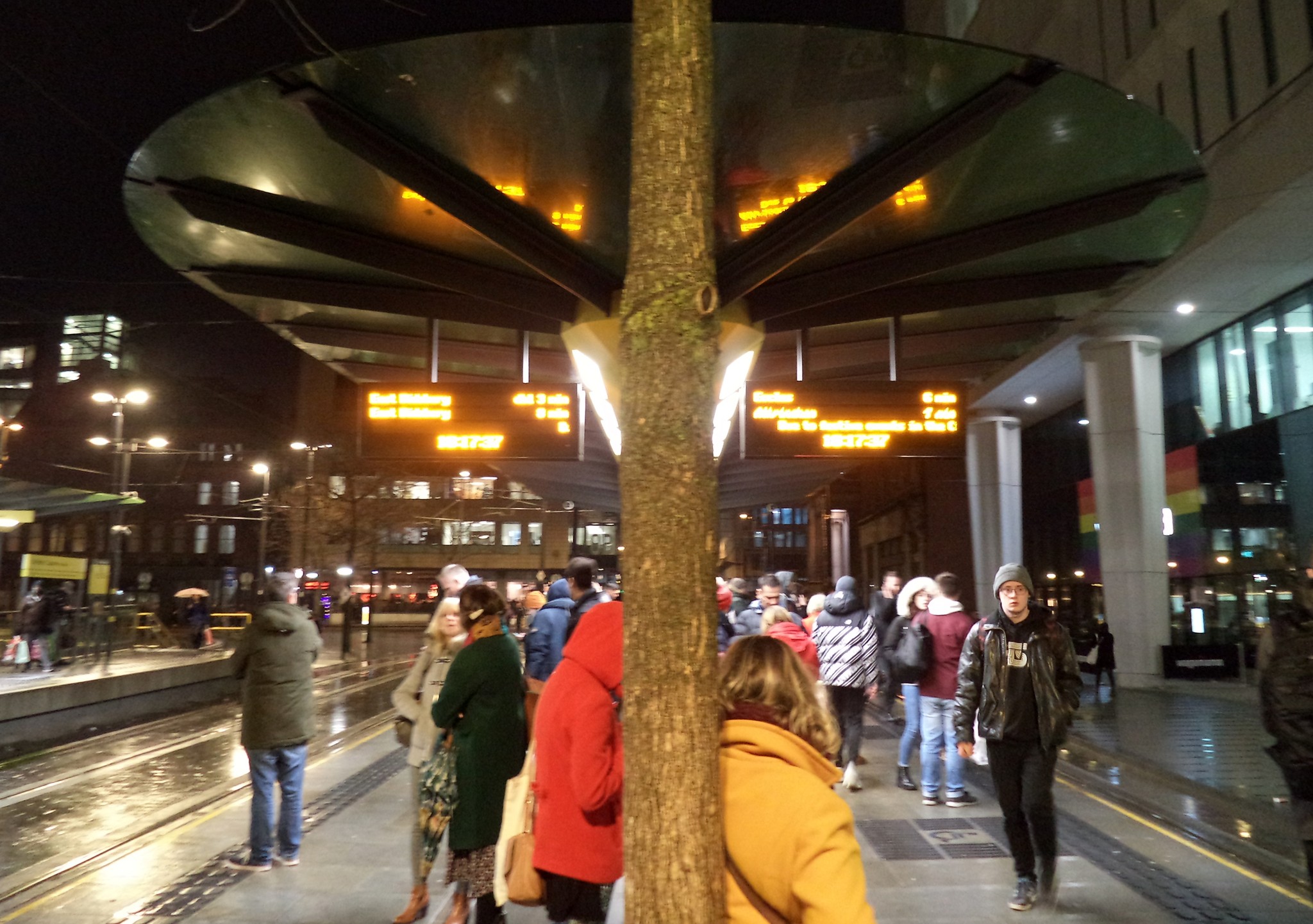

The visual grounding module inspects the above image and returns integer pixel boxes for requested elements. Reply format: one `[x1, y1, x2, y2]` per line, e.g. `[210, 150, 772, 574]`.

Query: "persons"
[1257, 578, 1313, 902]
[524, 557, 621, 746]
[953, 563, 1084, 912]
[13, 579, 77, 674]
[186, 594, 212, 650]
[533, 599, 626, 924]
[340, 595, 365, 659]
[228, 573, 323, 872]
[912, 574, 978, 807]
[812, 576, 880, 789]
[715, 571, 825, 681]
[431, 585, 528, 924]
[875, 573, 903, 720]
[1093, 622, 1115, 695]
[436, 564, 482, 596]
[883, 576, 939, 790]
[718, 636, 881, 924]
[392, 597, 474, 924]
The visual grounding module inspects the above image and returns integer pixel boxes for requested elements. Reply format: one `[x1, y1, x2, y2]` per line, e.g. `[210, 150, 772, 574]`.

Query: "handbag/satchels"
[504, 834, 547, 908]
[56, 619, 79, 649]
[1086, 646, 1099, 664]
[890, 625, 934, 682]
[3, 635, 21, 664]
[394, 691, 423, 748]
[204, 627, 214, 645]
[15, 640, 31, 664]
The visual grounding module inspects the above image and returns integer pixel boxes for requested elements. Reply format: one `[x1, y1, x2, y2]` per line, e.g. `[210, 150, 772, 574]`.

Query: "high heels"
[444, 895, 470, 924]
[396, 885, 429, 924]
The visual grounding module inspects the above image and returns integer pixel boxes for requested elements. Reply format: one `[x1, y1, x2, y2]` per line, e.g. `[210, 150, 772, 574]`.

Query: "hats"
[835, 575, 860, 593]
[525, 591, 547, 609]
[993, 563, 1034, 600]
[776, 572, 802, 586]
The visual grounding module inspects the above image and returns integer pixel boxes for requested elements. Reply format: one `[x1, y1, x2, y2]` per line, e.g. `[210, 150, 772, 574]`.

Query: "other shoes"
[836, 755, 866, 767]
[843, 761, 863, 790]
[41, 666, 53, 672]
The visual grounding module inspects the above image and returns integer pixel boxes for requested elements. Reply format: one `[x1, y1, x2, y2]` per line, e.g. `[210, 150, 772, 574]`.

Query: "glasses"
[1001, 585, 1026, 596]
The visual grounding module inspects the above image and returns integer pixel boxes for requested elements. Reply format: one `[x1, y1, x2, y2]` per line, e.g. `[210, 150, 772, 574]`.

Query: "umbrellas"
[173, 588, 210, 604]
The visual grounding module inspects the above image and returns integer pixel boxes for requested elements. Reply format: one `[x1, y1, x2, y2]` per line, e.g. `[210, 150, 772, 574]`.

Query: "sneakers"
[227, 851, 272, 872]
[272, 844, 299, 866]
[1009, 879, 1040, 910]
[946, 791, 978, 806]
[922, 796, 937, 806]
[897, 766, 915, 790]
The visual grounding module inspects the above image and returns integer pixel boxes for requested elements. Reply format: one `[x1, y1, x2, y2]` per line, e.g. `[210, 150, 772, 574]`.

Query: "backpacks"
[1261, 608, 1313, 754]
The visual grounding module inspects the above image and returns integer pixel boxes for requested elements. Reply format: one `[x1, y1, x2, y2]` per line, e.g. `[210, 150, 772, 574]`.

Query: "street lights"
[562, 499, 578, 561]
[251, 460, 272, 604]
[288, 441, 319, 583]
[83, 387, 172, 600]
